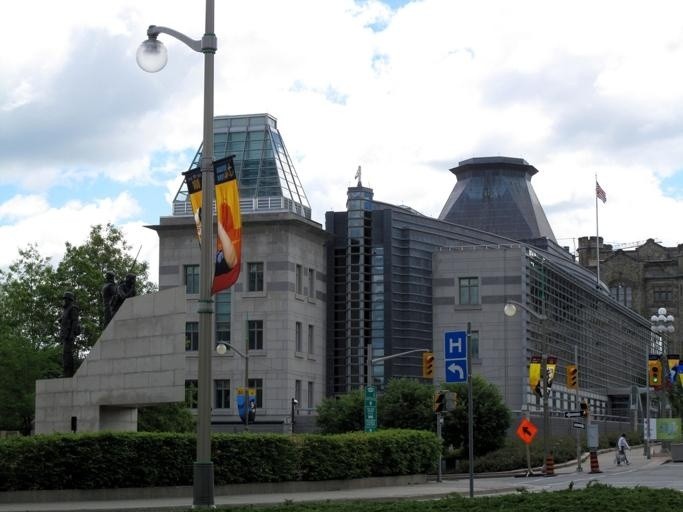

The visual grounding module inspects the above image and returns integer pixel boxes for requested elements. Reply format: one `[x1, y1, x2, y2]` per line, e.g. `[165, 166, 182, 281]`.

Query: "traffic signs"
[364, 386, 378, 432]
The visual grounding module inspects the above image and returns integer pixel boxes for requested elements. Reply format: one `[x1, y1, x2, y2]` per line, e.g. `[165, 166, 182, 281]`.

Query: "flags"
[247, 388, 257, 422]
[234, 386, 245, 425]
[185, 152, 244, 298]
[594, 173, 607, 204]
[545, 355, 557, 399]
[528, 355, 541, 400]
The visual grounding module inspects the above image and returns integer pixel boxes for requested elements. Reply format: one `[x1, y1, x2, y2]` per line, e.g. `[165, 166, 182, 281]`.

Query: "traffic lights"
[648, 355, 662, 385]
[579, 402, 588, 416]
[423, 353, 435, 379]
[567, 365, 577, 388]
[433, 393, 444, 412]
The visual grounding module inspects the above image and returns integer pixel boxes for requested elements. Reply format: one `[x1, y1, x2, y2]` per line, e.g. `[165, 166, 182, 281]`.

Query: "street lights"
[217, 341, 250, 426]
[651, 308, 675, 355]
[292, 397, 298, 433]
[504, 300, 548, 472]
[135, 0, 217, 510]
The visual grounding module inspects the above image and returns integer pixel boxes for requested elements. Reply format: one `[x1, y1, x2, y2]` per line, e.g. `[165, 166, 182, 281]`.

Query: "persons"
[615, 433, 632, 466]
